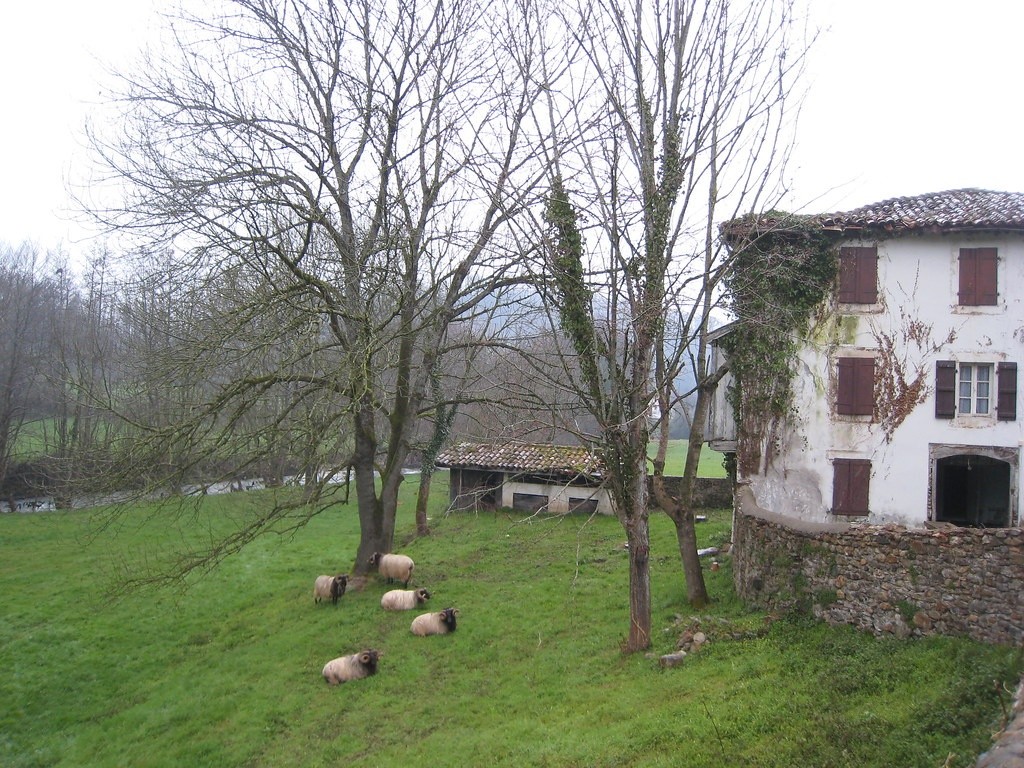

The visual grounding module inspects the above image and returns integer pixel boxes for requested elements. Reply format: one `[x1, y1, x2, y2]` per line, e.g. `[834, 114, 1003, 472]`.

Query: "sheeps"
[410, 607, 460, 637]
[322, 647, 384, 685]
[312, 574, 354, 605]
[380, 587, 436, 611]
[366, 551, 415, 589]
